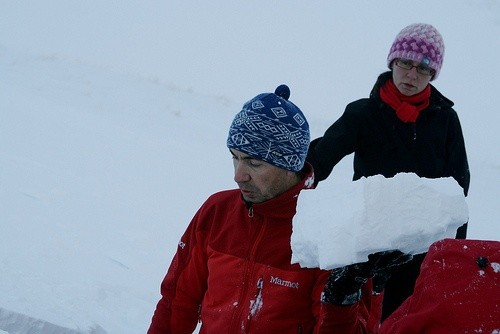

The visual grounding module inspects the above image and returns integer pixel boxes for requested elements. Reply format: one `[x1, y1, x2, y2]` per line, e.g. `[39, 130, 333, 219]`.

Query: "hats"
[227, 85, 310, 170]
[386, 23, 444, 82]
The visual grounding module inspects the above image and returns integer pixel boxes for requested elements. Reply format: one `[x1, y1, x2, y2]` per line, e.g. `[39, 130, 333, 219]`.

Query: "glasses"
[395, 58, 435, 75]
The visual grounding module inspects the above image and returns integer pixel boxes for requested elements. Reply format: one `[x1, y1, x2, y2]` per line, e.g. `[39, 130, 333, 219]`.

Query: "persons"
[310, 23, 471, 327]
[148, 85, 380, 334]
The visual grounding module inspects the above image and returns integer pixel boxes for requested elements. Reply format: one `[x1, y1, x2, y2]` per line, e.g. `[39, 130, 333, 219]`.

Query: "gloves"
[320, 251, 416, 308]
